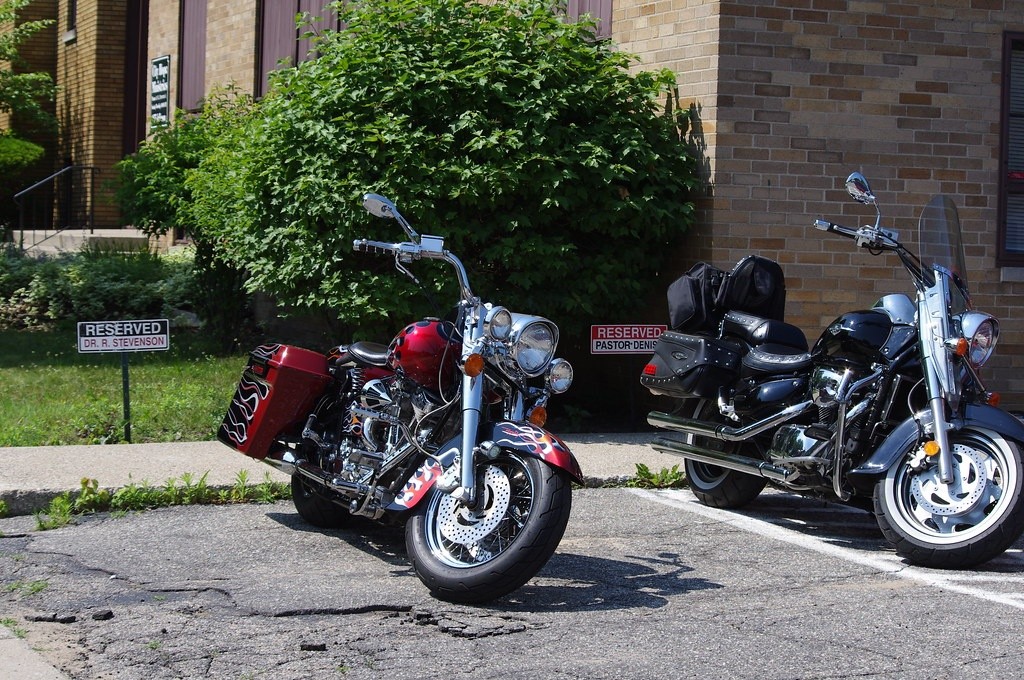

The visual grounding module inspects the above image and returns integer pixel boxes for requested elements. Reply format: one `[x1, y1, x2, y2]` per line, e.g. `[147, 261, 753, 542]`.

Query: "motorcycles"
[639, 171, 1024, 571]
[217, 193, 586, 604]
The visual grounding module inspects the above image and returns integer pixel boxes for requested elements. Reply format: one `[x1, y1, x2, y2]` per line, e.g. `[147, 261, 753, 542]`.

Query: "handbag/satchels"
[666, 254, 787, 334]
